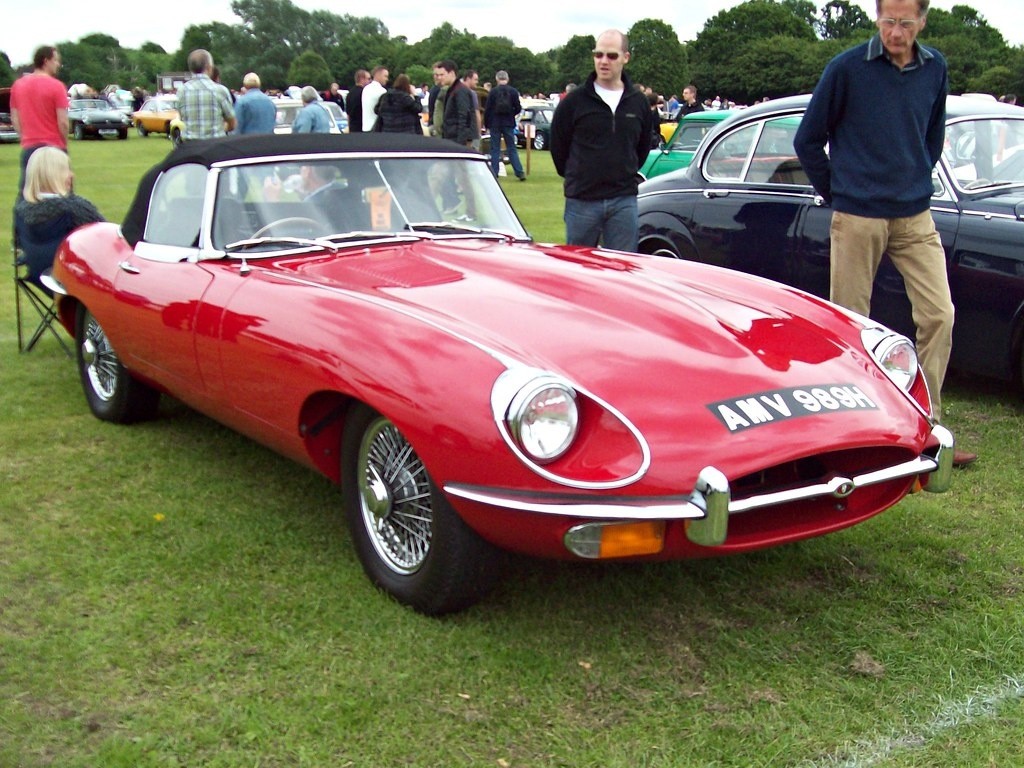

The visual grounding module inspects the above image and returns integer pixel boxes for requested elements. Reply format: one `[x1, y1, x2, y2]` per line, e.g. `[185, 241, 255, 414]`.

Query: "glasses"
[591, 50, 624, 60]
[875, 8, 922, 29]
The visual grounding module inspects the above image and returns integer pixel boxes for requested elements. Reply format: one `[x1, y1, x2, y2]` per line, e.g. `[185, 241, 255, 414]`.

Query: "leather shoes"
[953, 447, 977, 464]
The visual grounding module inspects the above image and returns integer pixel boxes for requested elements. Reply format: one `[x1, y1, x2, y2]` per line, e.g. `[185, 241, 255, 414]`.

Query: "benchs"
[214, 197, 342, 248]
[715, 162, 814, 185]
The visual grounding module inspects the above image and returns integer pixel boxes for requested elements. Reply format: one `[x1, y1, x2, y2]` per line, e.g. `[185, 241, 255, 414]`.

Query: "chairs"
[11, 211, 79, 357]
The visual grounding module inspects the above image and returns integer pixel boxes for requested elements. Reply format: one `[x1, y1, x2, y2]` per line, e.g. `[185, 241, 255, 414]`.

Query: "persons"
[792, 0, 977, 462]
[8, 28, 769, 291]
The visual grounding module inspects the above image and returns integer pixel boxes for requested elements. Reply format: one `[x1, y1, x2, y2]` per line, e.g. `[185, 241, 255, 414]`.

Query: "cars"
[659, 122, 679, 147]
[168, 115, 186, 149]
[513, 106, 557, 150]
[636, 93, 1024, 411]
[53, 131, 955, 617]
[0, 88, 20, 143]
[67, 100, 130, 140]
[130, 96, 180, 136]
[636, 110, 830, 185]
[105, 92, 134, 117]
[271, 100, 350, 135]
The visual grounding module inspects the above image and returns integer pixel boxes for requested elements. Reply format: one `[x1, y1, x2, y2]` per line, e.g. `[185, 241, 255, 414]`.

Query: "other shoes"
[519, 172, 527, 181]
[452, 216, 476, 222]
